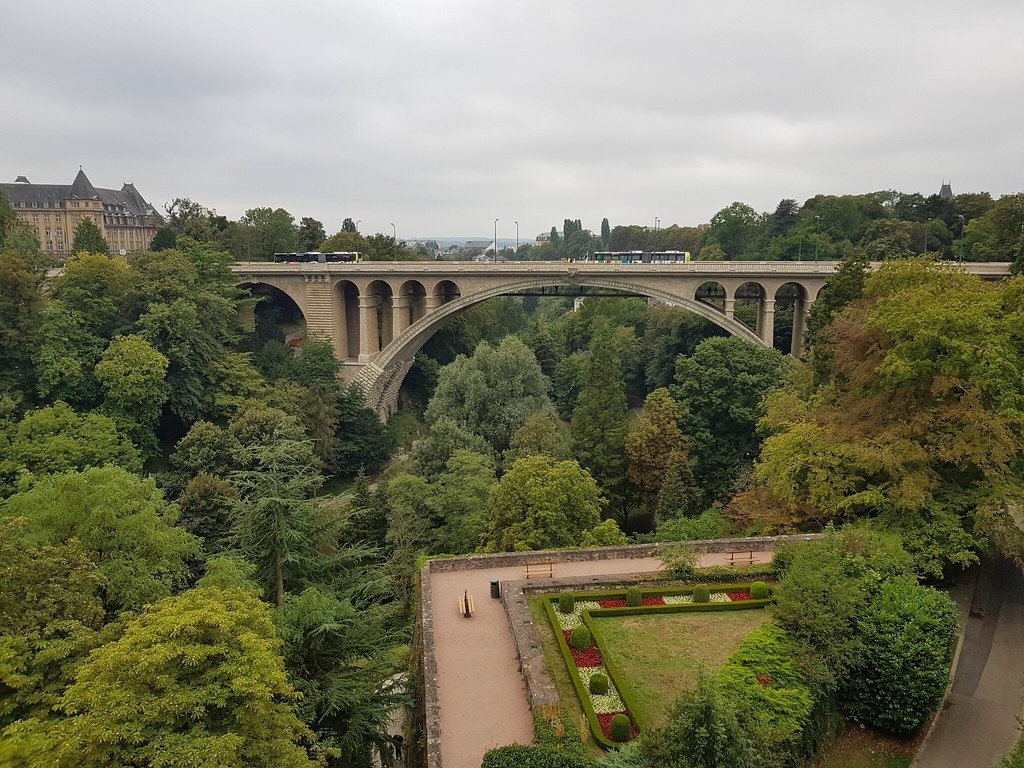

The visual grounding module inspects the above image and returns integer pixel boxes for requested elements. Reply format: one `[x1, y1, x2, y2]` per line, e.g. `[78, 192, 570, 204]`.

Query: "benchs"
[520, 562, 558, 579]
[459, 588, 476, 618]
[724, 550, 762, 564]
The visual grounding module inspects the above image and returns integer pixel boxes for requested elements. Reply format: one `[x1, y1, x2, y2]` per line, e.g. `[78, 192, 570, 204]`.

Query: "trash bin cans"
[490, 579, 499, 598]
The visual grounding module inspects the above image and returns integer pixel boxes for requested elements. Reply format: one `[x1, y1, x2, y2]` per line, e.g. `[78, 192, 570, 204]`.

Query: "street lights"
[924, 217, 932, 260]
[814, 216, 820, 263]
[657, 219, 661, 252]
[515, 221, 518, 250]
[494, 218, 499, 263]
[391, 223, 397, 262]
[355, 220, 362, 251]
[654, 216, 659, 264]
[798, 219, 806, 263]
[958, 214, 965, 267]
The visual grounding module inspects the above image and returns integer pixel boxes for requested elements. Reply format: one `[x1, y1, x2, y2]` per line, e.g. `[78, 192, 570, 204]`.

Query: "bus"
[274, 252, 363, 264]
[597, 252, 691, 265]
